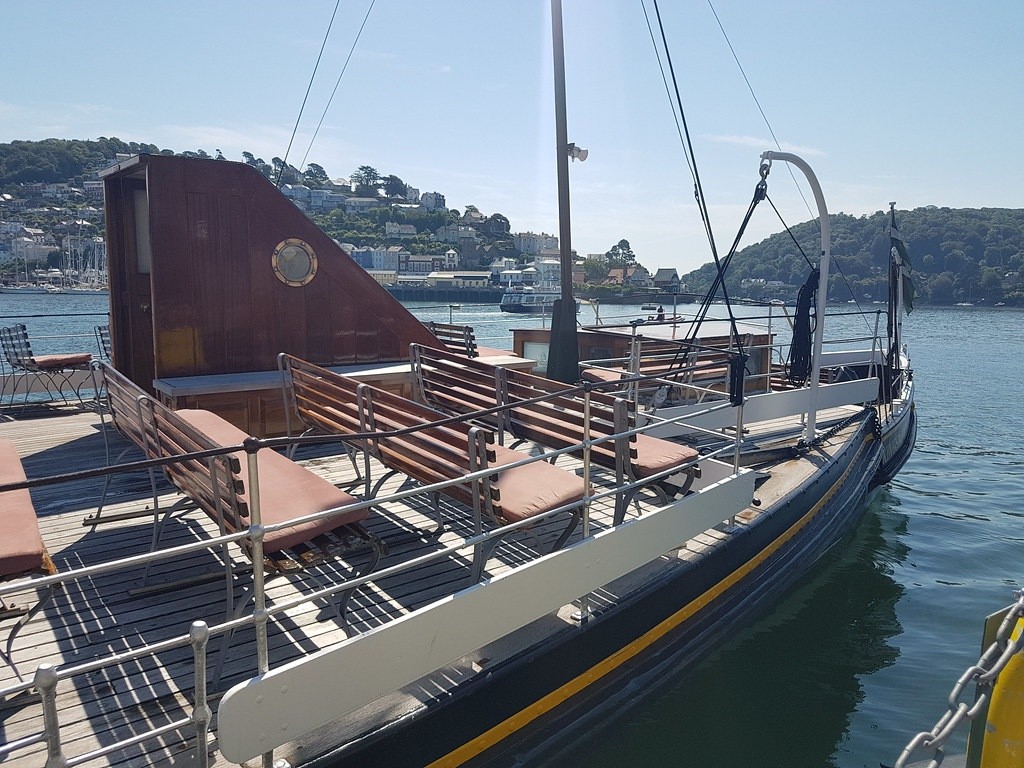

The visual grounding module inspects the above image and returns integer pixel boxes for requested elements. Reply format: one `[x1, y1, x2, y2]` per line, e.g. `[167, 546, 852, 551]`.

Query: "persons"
[658, 305, 664, 322]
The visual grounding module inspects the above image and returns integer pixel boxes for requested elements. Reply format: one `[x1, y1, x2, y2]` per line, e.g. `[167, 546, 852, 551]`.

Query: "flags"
[891, 217, 915, 316]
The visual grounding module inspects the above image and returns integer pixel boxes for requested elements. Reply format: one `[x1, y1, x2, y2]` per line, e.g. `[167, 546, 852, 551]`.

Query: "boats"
[498, 271, 582, 313]
[0, 2, 923, 768]
[627, 316, 684, 326]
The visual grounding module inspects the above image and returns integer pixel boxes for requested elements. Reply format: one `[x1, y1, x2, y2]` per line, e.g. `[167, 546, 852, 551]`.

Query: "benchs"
[277, 352, 596, 584]
[153, 358, 536, 411]
[409, 342, 701, 527]
[421, 322, 517, 357]
[84, 358, 390, 705]
[93, 326, 110, 400]
[0, 323, 95, 414]
[581, 334, 754, 403]
[636, 376, 881, 464]
[0, 438, 61, 710]
